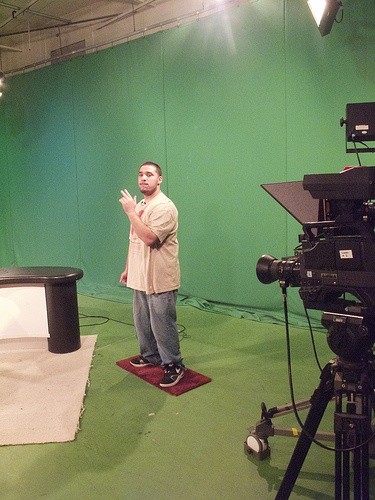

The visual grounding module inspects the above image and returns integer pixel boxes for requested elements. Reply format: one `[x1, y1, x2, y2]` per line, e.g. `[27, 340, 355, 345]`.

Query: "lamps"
[340, 102, 375, 153]
[306, 0, 343, 37]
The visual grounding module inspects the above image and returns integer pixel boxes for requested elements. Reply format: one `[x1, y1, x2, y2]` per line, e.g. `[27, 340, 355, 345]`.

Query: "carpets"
[0, 333, 98, 448]
[116, 353, 211, 396]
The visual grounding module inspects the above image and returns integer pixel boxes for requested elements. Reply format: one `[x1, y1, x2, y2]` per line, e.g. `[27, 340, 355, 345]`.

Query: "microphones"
[140, 201, 146, 204]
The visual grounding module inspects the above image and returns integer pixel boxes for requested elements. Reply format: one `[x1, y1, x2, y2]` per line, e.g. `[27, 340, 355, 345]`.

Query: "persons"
[119, 161, 186, 388]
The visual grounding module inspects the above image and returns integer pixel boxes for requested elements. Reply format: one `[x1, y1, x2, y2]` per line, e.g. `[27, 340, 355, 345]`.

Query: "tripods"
[273, 358, 375, 500]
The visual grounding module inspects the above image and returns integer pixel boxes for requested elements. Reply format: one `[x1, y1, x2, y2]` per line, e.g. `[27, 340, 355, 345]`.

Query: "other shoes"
[159, 366, 185, 387]
[130, 355, 162, 367]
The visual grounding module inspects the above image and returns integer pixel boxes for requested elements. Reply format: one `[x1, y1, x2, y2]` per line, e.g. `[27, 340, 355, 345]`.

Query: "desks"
[0, 267, 83, 354]
